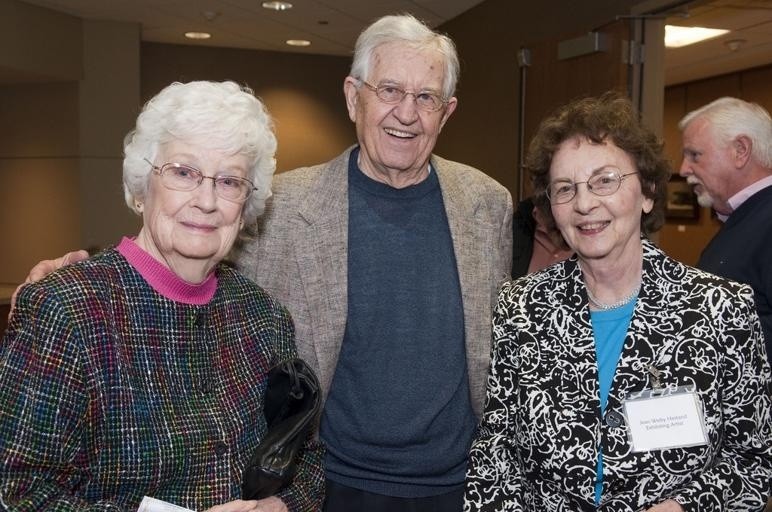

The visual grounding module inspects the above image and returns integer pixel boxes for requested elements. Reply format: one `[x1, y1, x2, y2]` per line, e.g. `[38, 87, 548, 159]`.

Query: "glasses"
[355, 76, 450, 112]
[543, 168, 643, 204]
[143, 157, 259, 202]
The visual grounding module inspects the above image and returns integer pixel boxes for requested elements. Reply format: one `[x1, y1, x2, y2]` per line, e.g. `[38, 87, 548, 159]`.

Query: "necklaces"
[583, 286, 643, 310]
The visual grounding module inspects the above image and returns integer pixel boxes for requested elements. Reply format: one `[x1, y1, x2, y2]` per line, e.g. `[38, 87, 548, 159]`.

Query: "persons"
[510, 195, 575, 282]
[7, 13, 516, 510]
[680, 96, 770, 368]
[461, 88, 772, 510]
[1, 77, 328, 511]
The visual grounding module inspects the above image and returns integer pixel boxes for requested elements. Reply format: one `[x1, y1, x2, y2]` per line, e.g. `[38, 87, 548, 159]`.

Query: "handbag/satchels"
[244, 357, 323, 500]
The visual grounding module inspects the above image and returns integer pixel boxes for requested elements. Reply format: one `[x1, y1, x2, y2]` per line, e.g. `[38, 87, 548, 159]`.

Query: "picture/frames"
[665, 174, 702, 224]
